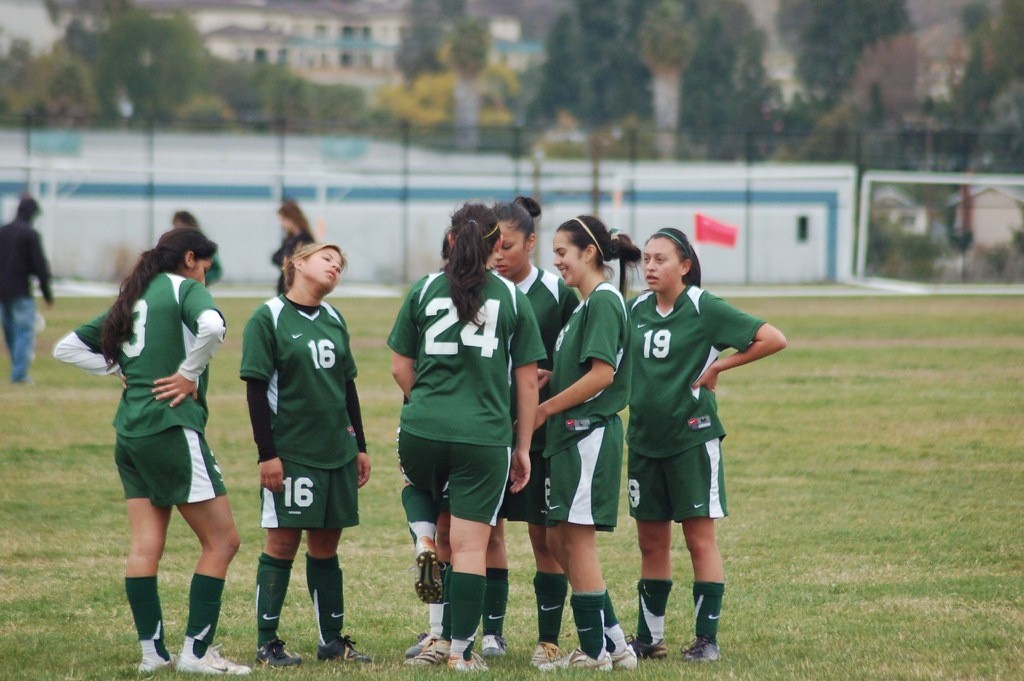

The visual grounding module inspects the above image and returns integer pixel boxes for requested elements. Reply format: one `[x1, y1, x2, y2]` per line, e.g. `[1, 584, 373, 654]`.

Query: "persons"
[239, 241, 371, 668]
[387, 196, 637, 672]
[271, 200, 315, 299]
[626, 227, 787, 663]
[53, 227, 253, 678]
[166, 211, 222, 294]
[1, 196, 57, 384]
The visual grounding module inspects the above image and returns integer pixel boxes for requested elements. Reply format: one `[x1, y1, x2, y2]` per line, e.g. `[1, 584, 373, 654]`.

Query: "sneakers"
[481, 635, 508, 656]
[316, 635, 372, 663]
[405, 633, 439, 658]
[531, 642, 561, 667]
[625, 634, 668, 660]
[175, 643, 252, 676]
[255, 636, 302, 668]
[446, 651, 489, 671]
[681, 635, 722, 662]
[402, 638, 452, 667]
[413, 548, 447, 605]
[137, 652, 178, 673]
[537, 648, 613, 672]
[610, 644, 638, 669]
